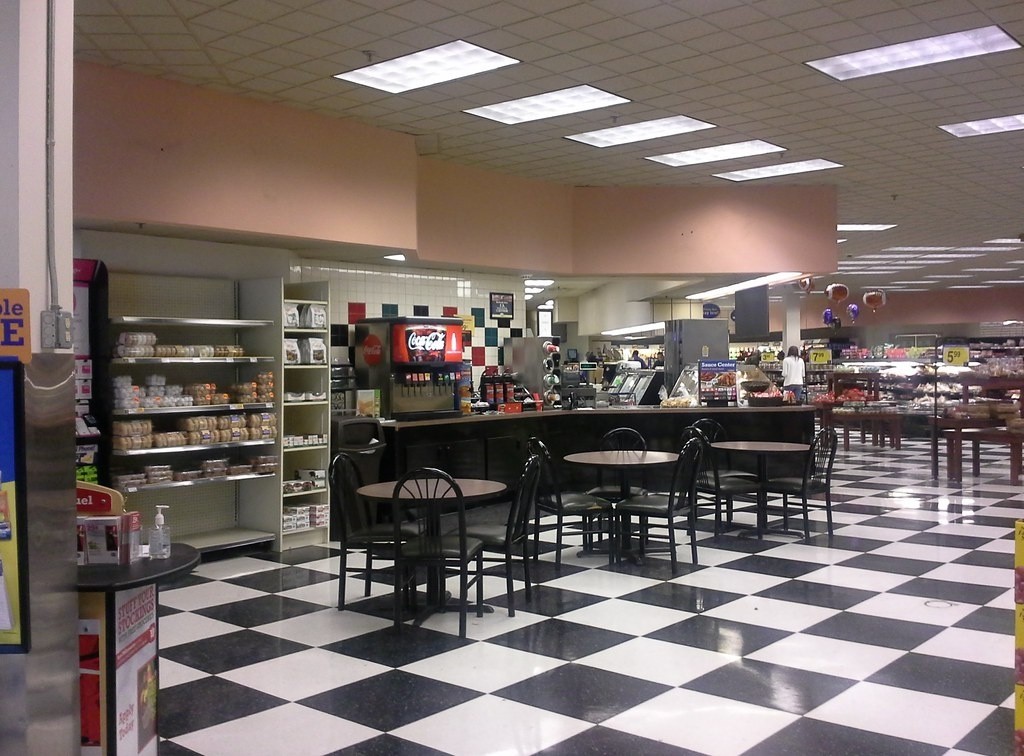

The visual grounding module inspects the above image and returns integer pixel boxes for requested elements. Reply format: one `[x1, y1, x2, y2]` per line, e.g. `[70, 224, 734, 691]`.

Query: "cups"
[547, 344, 560, 353]
[404, 328, 447, 362]
[548, 377, 559, 384]
[549, 394, 560, 401]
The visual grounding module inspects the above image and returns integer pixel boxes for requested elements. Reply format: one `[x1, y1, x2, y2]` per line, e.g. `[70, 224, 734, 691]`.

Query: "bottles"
[451, 332, 456, 351]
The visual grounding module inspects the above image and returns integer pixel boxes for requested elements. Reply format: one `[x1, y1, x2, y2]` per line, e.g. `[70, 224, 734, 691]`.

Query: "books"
[76, 509, 142, 566]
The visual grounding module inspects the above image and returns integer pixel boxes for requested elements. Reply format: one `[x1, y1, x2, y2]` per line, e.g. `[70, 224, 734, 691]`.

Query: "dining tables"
[356, 478, 508, 629]
[710, 441, 811, 539]
[564, 450, 680, 567]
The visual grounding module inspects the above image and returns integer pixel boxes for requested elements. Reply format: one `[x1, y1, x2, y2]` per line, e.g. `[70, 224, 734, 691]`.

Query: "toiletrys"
[148, 504, 171, 559]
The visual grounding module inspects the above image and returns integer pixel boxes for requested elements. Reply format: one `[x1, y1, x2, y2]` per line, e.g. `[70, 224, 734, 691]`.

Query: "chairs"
[615, 437, 704, 574]
[528, 436, 614, 571]
[680, 426, 763, 543]
[392, 467, 484, 638]
[584, 427, 648, 550]
[451, 454, 540, 618]
[692, 418, 759, 529]
[328, 452, 427, 612]
[765, 426, 838, 539]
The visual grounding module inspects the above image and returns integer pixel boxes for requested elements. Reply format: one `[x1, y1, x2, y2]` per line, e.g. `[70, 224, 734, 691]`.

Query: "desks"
[381, 403, 815, 525]
[815, 373, 1024, 487]
[77, 542, 202, 756]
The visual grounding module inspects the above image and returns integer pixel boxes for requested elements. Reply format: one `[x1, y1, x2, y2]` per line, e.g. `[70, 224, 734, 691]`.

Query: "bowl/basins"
[740, 381, 770, 393]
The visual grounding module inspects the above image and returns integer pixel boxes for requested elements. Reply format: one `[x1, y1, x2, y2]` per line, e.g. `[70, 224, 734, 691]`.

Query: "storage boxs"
[76, 515, 122, 565]
[283, 303, 328, 532]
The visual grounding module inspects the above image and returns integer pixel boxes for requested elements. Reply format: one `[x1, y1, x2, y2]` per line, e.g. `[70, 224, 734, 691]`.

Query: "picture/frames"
[489, 292, 514, 319]
[1, 358, 33, 654]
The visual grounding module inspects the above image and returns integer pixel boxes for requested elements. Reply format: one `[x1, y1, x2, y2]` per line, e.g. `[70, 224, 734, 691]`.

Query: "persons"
[782, 346, 806, 401]
[628, 350, 648, 369]
[652, 353, 664, 370]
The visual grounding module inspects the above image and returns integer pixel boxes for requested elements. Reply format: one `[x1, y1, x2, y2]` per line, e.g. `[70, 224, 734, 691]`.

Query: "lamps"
[683, 272, 803, 301]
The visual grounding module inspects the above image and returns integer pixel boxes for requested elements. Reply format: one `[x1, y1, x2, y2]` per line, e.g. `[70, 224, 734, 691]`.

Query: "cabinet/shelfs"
[110, 315, 277, 555]
[238, 278, 331, 551]
[623, 335, 1024, 439]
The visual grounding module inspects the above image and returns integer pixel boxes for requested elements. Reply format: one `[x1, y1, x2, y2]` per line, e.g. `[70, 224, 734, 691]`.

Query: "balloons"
[846, 304, 859, 320]
[822, 309, 841, 333]
